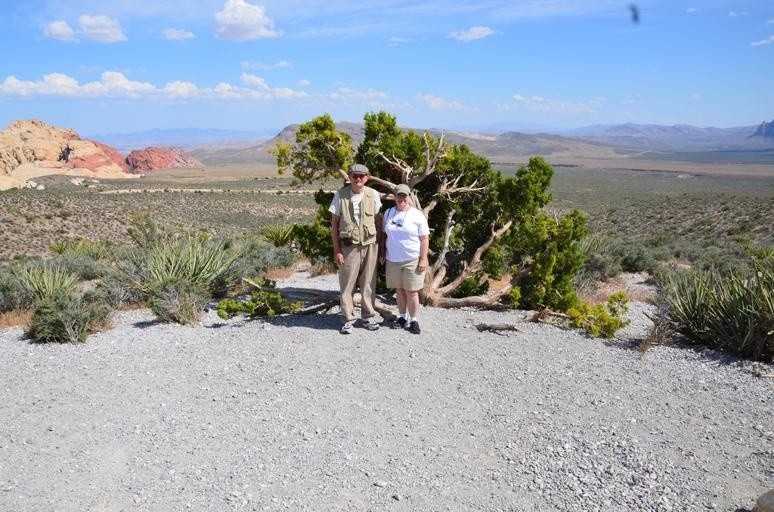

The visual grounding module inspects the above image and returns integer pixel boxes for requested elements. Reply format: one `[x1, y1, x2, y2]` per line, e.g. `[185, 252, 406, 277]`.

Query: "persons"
[326, 163, 384, 335]
[380, 183, 430, 336]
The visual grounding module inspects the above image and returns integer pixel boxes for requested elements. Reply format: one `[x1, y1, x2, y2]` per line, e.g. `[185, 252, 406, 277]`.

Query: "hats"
[394, 184, 411, 196]
[349, 164, 369, 176]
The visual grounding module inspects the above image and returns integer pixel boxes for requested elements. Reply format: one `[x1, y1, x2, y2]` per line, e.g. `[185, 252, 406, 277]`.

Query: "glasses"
[392, 221, 402, 226]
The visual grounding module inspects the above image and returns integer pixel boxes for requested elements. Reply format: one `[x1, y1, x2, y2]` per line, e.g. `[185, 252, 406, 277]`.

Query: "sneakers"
[361, 320, 379, 331]
[341, 322, 353, 334]
[409, 321, 420, 334]
[393, 317, 408, 328]
